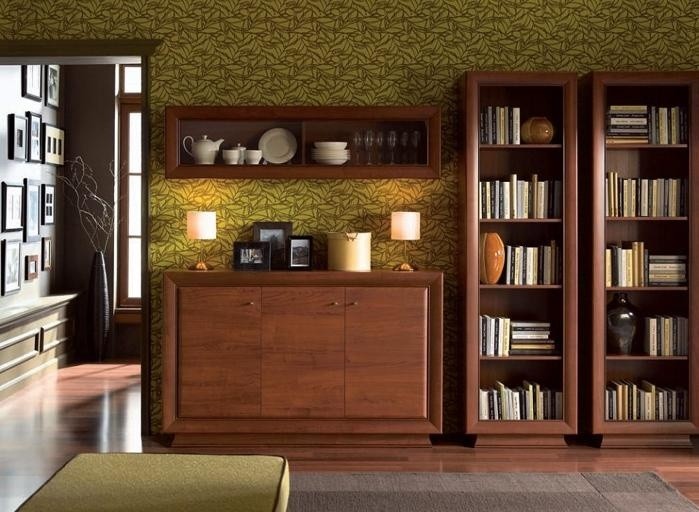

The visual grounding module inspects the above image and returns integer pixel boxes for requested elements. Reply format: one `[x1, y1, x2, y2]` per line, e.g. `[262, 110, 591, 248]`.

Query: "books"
[507, 240, 562, 285]
[606, 241, 687, 287]
[643, 315, 689, 356]
[605, 172, 688, 217]
[479, 314, 556, 357]
[605, 104, 688, 145]
[479, 174, 561, 220]
[478, 106, 520, 145]
[479, 380, 562, 421]
[606, 377, 690, 421]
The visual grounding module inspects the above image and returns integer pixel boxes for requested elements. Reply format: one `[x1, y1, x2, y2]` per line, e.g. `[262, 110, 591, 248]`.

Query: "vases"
[87, 251, 110, 362]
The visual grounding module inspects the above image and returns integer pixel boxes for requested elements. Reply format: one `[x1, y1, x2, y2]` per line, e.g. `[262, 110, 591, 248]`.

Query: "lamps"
[186, 211, 217, 270]
[391, 212, 420, 270]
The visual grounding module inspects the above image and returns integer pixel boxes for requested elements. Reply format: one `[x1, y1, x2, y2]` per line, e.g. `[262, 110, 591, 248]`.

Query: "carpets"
[286, 471, 698, 511]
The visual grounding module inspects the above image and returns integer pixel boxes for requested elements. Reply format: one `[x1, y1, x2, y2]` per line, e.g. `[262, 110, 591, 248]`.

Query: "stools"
[15, 451, 290, 512]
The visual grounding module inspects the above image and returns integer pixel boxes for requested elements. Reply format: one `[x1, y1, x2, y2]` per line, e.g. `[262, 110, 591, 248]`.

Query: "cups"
[327, 231, 372, 271]
[244, 150, 262, 166]
[220, 150, 241, 165]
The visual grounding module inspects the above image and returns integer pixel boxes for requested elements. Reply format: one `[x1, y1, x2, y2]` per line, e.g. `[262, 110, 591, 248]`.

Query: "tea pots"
[183, 133, 224, 165]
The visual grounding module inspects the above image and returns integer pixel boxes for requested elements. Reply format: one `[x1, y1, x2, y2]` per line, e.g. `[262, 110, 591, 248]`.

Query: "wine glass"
[352, 130, 423, 165]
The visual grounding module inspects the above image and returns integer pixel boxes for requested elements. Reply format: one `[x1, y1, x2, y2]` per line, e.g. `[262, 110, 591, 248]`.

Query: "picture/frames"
[1, 66, 65, 295]
[234, 240, 271, 269]
[254, 222, 292, 269]
[285, 235, 313, 269]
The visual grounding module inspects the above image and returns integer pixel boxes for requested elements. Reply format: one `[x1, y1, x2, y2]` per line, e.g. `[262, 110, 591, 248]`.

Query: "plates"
[310, 141, 350, 165]
[258, 128, 297, 164]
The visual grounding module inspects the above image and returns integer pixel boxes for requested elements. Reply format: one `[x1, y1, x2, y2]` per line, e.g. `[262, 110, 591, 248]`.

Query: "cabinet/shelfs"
[165, 105, 441, 179]
[162, 270, 445, 451]
[572, 69, 699, 448]
[457, 69, 578, 449]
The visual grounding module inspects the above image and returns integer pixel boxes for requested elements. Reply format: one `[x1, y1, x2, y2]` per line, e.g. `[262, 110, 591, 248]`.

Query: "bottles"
[479, 231, 506, 285]
[607, 293, 641, 354]
[521, 116, 555, 144]
[232, 143, 247, 165]
